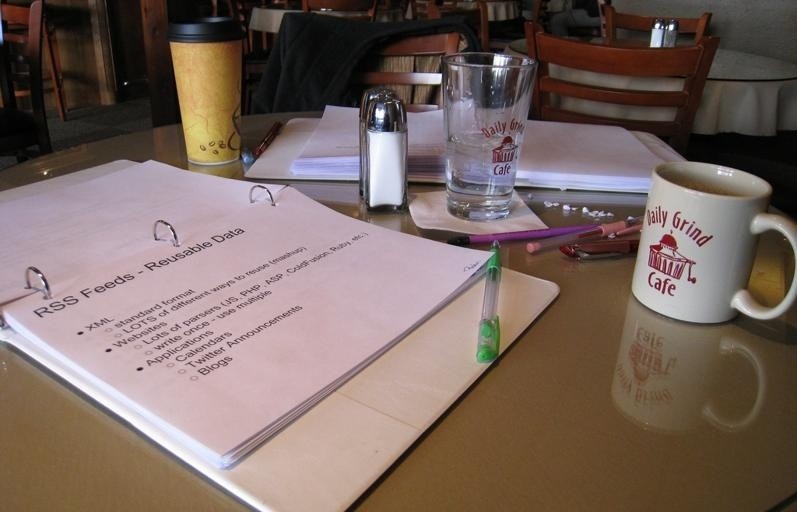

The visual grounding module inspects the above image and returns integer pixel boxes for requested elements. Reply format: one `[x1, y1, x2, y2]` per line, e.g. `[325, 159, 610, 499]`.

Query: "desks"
[505, 34, 781, 146]
[0, 108, 797, 510]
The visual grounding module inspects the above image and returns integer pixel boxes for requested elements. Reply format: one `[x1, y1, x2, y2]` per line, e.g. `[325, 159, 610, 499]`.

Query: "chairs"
[0, 0, 70, 164]
[304, 2, 721, 142]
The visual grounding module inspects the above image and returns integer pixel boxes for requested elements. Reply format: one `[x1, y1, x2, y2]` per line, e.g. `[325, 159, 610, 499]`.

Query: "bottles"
[648, 17, 680, 48]
[356, 83, 414, 216]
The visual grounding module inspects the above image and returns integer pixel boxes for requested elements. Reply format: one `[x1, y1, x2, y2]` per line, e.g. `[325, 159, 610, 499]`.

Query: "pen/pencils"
[256, 120, 282, 156]
[442, 218, 642, 262]
[479, 240, 502, 362]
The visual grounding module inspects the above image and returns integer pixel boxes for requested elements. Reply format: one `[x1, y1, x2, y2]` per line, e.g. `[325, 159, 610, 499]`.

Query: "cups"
[165, 16, 248, 166]
[629, 160, 796, 326]
[609, 291, 770, 438]
[439, 52, 539, 224]
